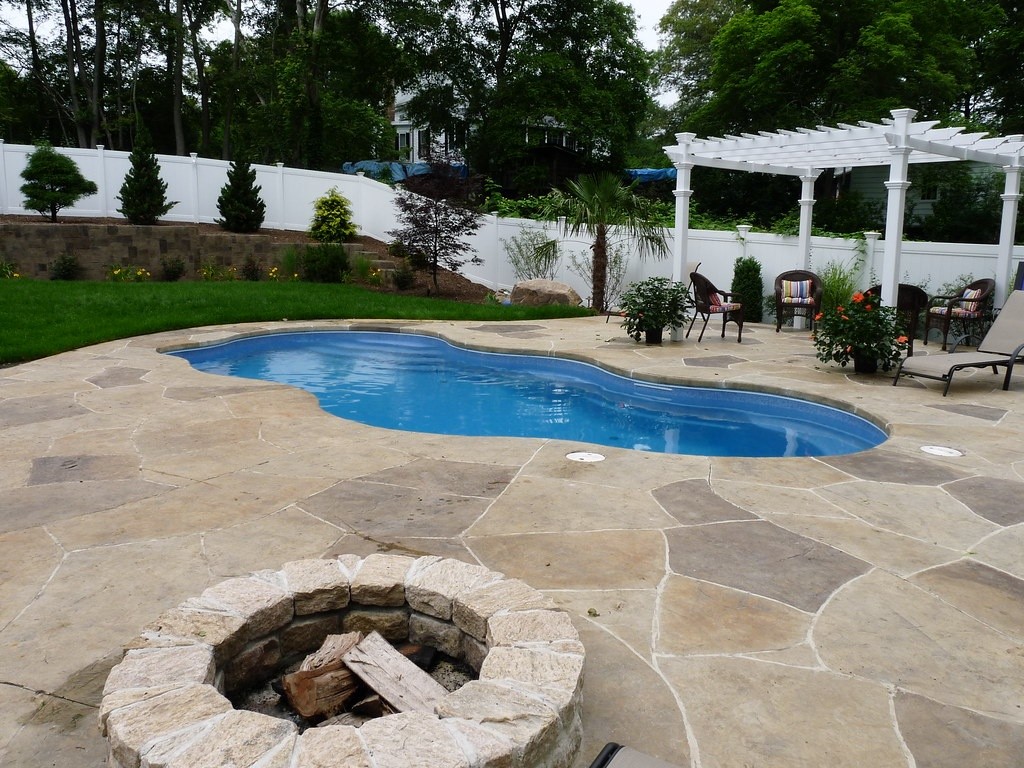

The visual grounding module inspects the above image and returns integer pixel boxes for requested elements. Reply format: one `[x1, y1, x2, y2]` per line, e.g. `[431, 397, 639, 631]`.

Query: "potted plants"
[606, 275, 698, 342]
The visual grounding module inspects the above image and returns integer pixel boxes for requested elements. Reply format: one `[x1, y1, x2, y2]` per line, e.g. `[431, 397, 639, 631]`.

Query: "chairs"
[923, 279, 995, 350]
[892, 290, 1024, 397]
[774, 270, 822, 335]
[686, 272, 747, 344]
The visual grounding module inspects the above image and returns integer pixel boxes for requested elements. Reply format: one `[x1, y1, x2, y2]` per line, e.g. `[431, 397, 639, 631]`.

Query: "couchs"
[863, 284, 928, 340]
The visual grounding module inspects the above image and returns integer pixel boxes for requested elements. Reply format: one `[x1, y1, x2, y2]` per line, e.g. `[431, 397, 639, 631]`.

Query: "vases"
[854, 356, 877, 374]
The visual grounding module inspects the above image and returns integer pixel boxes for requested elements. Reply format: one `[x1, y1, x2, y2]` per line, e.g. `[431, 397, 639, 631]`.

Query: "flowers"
[808, 291, 911, 373]
